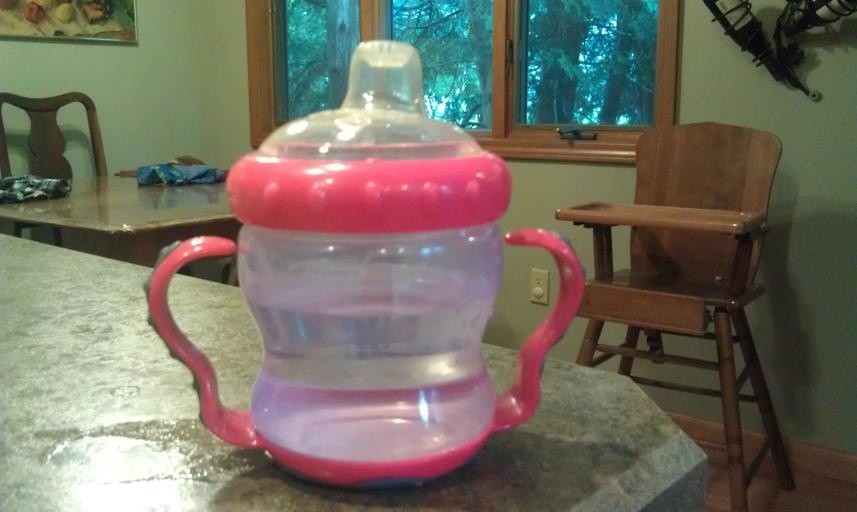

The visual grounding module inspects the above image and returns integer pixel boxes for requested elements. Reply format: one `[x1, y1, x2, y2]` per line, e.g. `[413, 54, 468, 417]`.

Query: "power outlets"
[530, 268, 549, 305]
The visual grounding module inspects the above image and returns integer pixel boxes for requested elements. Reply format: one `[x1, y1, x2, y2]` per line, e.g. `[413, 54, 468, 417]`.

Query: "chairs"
[0, 89, 107, 250]
[553, 120, 797, 512]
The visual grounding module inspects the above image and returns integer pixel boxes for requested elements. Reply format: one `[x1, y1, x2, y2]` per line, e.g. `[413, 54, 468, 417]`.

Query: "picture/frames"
[0, 0, 137, 46]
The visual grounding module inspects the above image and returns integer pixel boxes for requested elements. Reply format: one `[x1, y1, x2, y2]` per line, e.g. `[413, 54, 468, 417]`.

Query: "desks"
[1, 172, 241, 287]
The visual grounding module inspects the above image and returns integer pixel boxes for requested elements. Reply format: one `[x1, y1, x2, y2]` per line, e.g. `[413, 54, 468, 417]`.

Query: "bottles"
[141, 39, 588, 490]
